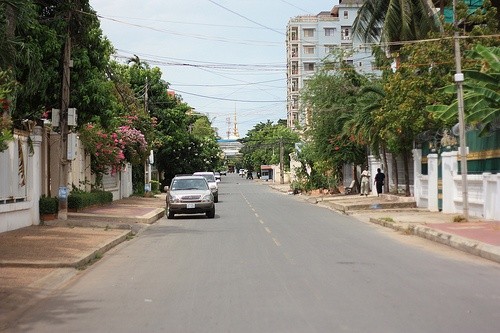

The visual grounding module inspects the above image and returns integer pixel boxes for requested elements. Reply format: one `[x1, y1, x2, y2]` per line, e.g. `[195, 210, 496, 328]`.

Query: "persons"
[375, 168, 385, 197]
[359, 165, 371, 196]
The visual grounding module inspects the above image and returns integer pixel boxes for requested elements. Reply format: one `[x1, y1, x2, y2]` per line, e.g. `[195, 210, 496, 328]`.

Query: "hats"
[365, 165, 369, 168]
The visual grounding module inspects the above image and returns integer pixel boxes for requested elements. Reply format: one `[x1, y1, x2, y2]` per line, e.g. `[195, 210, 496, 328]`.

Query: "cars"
[191, 171, 219, 203]
[220, 170, 226, 176]
[214, 172, 221, 181]
[239, 168, 260, 181]
[164, 175, 216, 219]
